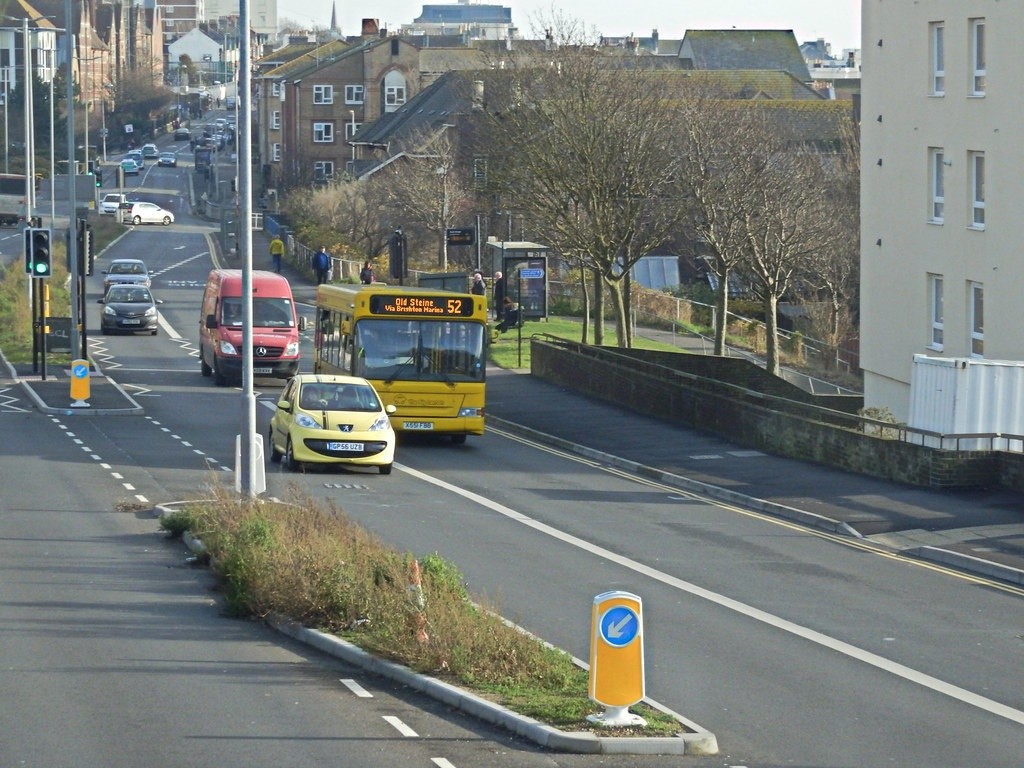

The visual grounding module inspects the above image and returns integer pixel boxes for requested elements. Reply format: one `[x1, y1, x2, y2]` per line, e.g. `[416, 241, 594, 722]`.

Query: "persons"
[113, 264, 140, 274]
[360, 261, 375, 284]
[470, 273, 486, 296]
[495, 297, 518, 334]
[312, 244, 332, 285]
[269, 235, 284, 273]
[304, 389, 328, 408]
[492, 271, 503, 321]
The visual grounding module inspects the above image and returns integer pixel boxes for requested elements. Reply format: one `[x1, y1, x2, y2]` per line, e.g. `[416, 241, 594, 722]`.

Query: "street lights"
[2, 13, 58, 224]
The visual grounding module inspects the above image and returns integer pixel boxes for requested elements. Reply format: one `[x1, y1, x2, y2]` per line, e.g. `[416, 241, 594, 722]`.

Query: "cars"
[101, 259, 154, 292]
[175, 128, 191, 139]
[99, 194, 127, 215]
[116, 202, 174, 225]
[206, 115, 236, 151]
[158, 152, 177, 166]
[96, 284, 165, 335]
[121, 159, 139, 175]
[268, 375, 396, 474]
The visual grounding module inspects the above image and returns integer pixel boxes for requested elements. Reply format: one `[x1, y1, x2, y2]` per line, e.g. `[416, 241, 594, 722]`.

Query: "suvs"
[142, 144, 159, 157]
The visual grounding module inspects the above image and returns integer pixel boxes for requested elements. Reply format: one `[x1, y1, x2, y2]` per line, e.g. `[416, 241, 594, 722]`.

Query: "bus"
[315, 284, 488, 444]
[125, 150, 146, 168]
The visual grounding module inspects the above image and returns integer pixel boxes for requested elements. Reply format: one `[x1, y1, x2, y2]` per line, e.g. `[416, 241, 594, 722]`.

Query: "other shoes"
[494, 317, 503, 322]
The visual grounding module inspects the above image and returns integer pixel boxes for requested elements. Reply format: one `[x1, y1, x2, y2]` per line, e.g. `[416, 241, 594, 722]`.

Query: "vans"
[200, 269, 300, 386]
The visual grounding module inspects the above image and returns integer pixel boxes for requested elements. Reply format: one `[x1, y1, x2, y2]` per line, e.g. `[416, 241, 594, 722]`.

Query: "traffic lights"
[88, 161, 94, 176]
[94, 168, 103, 187]
[31, 228, 51, 277]
